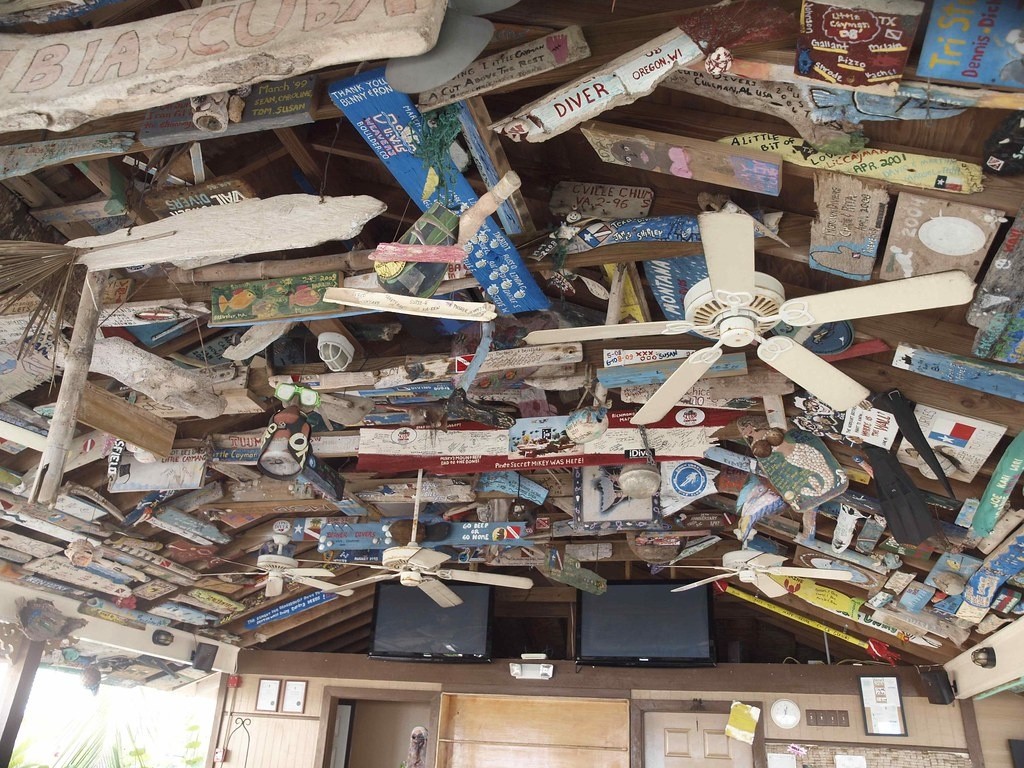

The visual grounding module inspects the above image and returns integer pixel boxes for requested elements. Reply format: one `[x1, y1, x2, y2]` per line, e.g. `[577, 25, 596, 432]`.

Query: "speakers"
[192, 642, 219, 672]
[920, 670, 955, 705]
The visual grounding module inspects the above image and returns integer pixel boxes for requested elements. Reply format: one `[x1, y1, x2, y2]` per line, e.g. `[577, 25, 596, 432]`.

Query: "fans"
[193, 210, 975, 607]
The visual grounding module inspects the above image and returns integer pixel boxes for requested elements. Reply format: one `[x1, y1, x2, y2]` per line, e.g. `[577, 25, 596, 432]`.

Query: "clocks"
[770, 698, 800, 729]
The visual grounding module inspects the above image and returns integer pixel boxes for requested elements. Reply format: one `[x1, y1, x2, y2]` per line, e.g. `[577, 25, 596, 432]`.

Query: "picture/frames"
[255, 678, 282, 712]
[572, 466, 661, 531]
[281, 680, 308, 714]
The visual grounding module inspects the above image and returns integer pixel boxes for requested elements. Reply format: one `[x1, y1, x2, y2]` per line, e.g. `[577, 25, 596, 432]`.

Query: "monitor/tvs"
[369, 580, 493, 664]
[575, 578, 717, 668]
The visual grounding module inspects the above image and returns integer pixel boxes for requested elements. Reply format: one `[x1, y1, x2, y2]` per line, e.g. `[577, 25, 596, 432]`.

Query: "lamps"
[617, 462, 661, 498]
[970, 647, 996, 669]
[317, 331, 355, 372]
[257, 394, 313, 481]
[151, 630, 174, 646]
